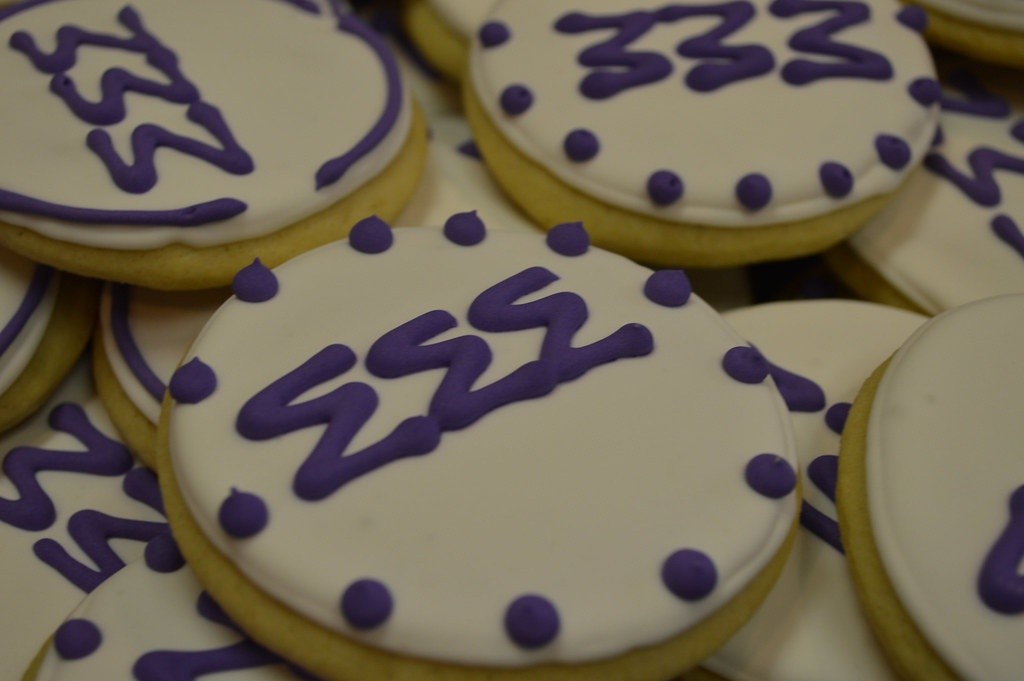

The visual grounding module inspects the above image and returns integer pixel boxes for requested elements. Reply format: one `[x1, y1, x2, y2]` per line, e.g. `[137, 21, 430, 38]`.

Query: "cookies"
[0, 0, 1024, 681]
